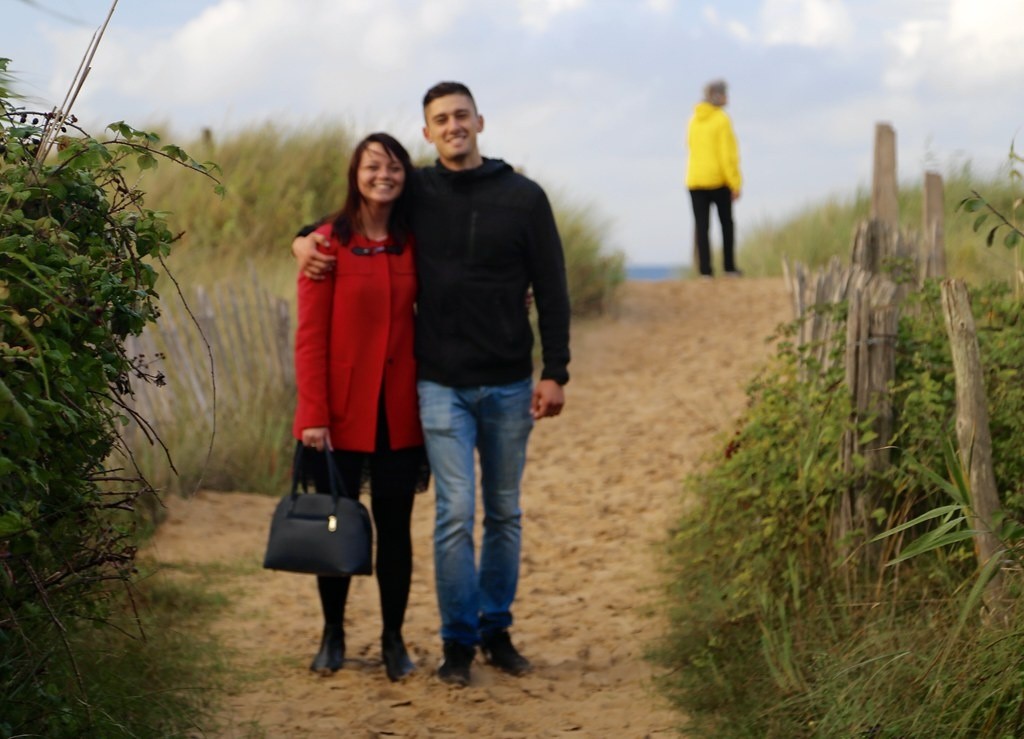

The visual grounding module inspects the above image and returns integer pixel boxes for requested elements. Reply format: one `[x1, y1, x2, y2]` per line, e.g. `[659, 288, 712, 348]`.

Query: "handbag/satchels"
[264, 439, 374, 576]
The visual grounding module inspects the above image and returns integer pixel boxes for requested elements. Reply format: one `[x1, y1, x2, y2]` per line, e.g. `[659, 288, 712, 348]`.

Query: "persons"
[687, 81, 741, 276]
[287, 81, 572, 682]
[292, 131, 430, 682]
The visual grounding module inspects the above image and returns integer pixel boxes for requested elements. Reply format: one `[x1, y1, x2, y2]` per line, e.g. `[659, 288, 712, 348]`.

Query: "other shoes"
[380, 634, 415, 681]
[481, 633, 531, 677]
[438, 646, 477, 686]
[726, 270, 743, 279]
[311, 626, 346, 675]
